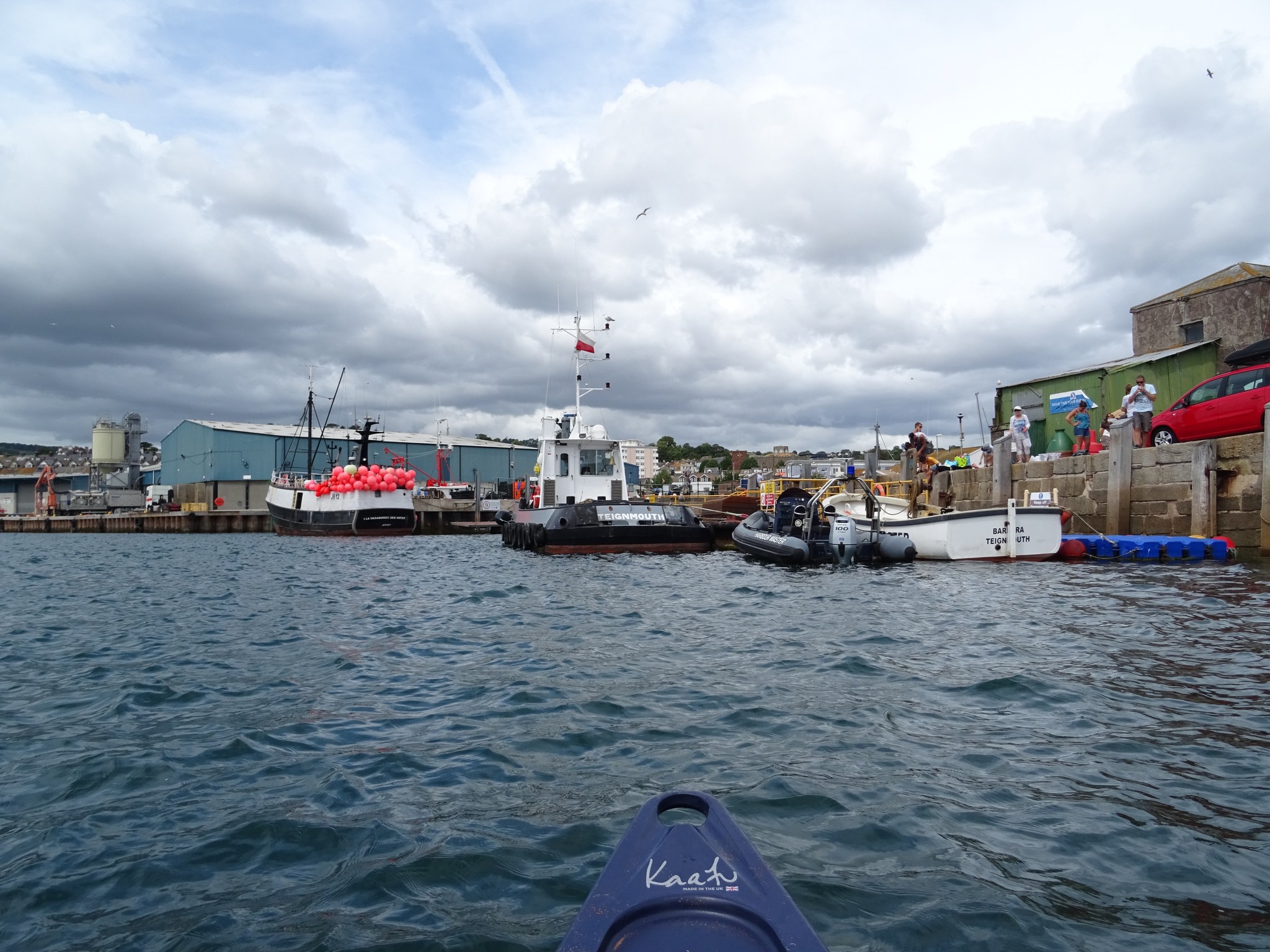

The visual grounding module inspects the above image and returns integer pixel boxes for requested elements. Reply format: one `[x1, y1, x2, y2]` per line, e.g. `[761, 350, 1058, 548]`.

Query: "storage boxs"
[499, 481, 525, 500]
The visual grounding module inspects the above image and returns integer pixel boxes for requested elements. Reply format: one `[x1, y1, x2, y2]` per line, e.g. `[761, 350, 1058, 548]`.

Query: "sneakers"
[1134, 444, 1142, 448]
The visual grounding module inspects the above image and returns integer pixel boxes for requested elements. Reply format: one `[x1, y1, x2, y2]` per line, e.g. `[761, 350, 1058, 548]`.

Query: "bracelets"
[1144, 390, 1147, 393]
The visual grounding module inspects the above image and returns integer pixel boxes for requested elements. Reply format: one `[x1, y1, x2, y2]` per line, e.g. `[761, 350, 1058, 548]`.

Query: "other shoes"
[983, 465, 988, 468]
[1016, 461, 1023, 463]
[989, 465, 993, 467]
[973, 463, 979, 468]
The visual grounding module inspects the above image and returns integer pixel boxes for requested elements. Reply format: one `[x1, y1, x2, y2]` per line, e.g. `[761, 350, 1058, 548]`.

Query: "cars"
[1143, 360, 1270, 447]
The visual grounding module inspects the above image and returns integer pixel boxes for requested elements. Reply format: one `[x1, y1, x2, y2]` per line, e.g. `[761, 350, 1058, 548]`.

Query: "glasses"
[1014, 409, 1020, 412]
[1136, 381, 1144, 384]
[1107, 415, 1111, 418]
[918, 426, 923, 428]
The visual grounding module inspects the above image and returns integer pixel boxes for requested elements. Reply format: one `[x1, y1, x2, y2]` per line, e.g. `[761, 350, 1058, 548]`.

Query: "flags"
[575, 331, 596, 354]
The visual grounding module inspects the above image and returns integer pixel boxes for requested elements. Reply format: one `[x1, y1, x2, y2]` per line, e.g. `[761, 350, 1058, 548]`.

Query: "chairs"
[783, 503, 806, 536]
[811, 504, 836, 539]
[1231, 383, 1244, 393]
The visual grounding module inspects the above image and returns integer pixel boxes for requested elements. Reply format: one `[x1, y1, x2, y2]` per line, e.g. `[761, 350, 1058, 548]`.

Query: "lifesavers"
[529, 487, 533, 507]
[44, 518, 49, 524]
[532, 486, 540, 508]
[281, 474, 287, 484]
[71, 519, 76, 527]
[134, 517, 143, 526]
[18, 518, 22, 525]
[872, 484, 886, 496]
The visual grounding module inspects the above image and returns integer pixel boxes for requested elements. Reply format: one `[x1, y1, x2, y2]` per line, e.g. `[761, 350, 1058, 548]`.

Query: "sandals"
[917, 470, 922, 473]
[922, 469, 927, 472]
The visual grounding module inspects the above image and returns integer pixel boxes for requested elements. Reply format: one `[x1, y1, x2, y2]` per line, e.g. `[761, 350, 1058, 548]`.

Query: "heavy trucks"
[143, 484, 180, 513]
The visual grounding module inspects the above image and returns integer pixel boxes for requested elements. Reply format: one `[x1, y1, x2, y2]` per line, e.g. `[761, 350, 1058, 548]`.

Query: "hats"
[1014, 406, 1022, 410]
[981, 443, 990, 452]
[931, 464, 937, 474]
[908, 432, 914, 441]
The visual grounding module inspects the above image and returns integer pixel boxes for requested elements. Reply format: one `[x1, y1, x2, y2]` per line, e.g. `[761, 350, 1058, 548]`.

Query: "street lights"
[936, 434, 943, 461]
[957, 413, 964, 455]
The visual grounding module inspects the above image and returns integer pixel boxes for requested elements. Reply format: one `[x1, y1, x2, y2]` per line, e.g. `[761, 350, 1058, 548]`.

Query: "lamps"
[613, 442, 618, 447]
[556, 441, 560, 445]
[608, 450, 620, 465]
[286, 462, 291, 465]
[243, 462, 247, 466]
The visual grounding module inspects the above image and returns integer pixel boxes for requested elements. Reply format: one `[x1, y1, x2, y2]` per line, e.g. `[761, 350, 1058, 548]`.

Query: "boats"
[730, 480, 918, 568]
[820, 406, 1066, 564]
[263, 362, 420, 540]
[494, 280, 717, 556]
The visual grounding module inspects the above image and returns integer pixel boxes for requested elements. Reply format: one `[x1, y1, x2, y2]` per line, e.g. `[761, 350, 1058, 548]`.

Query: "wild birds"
[636, 207, 652, 220]
[1207, 68, 1213, 79]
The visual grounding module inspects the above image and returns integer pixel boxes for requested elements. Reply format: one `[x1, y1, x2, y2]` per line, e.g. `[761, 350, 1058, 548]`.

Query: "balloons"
[306, 464, 416, 497]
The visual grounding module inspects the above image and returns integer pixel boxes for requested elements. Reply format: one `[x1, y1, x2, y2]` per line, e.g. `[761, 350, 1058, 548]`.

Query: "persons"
[1009, 406, 1031, 464]
[1120, 384, 1142, 449]
[1099, 413, 1113, 450]
[1128, 375, 1157, 449]
[1065, 400, 1091, 452]
[979, 443, 993, 468]
[757, 470, 763, 488]
[931, 463, 979, 474]
[901, 422, 929, 474]
[919, 456, 941, 486]
